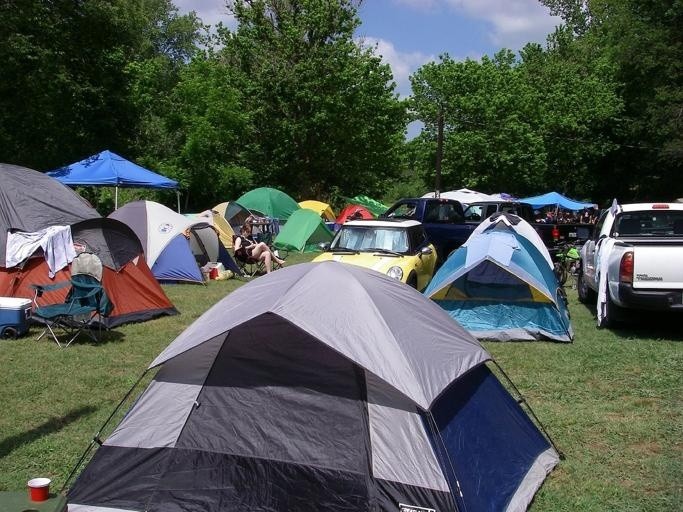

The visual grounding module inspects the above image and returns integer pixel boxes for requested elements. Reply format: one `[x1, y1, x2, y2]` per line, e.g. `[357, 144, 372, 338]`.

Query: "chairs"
[230, 232, 285, 280]
[27, 252, 102, 348]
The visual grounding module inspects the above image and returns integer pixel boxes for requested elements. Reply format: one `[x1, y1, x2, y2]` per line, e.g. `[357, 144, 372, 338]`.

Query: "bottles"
[265, 217, 269, 223]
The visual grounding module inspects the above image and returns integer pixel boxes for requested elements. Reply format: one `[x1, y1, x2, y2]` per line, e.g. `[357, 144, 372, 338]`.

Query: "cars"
[309, 218, 438, 291]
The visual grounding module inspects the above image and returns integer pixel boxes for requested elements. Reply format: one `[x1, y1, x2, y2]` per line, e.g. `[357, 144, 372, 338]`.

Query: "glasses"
[246, 230, 252, 233]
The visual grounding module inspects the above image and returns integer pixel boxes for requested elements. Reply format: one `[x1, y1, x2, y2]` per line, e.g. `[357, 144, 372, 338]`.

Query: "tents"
[0, 150, 245, 332]
[216, 187, 336, 253]
[334, 203, 374, 234]
[423, 211, 575, 344]
[491, 192, 598, 213]
[64, 260, 564, 512]
[420, 188, 508, 203]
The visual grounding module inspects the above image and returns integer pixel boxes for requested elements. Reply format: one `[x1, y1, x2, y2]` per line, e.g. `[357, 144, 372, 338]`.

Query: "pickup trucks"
[576, 198, 682, 329]
[376, 198, 545, 266]
[463, 201, 594, 264]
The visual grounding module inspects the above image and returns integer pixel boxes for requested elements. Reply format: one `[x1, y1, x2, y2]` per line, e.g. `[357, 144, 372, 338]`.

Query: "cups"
[260, 218, 265, 222]
[26, 477, 51, 502]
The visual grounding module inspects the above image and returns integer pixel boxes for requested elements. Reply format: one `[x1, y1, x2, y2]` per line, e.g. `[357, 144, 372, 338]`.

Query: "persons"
[235, 222, 286, 274]
[538, 209, 600, 224]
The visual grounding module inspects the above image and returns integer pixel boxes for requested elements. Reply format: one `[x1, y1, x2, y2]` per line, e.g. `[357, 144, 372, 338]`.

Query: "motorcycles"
[343, 209, 363, 222]
[552, 237, 579, 286]
